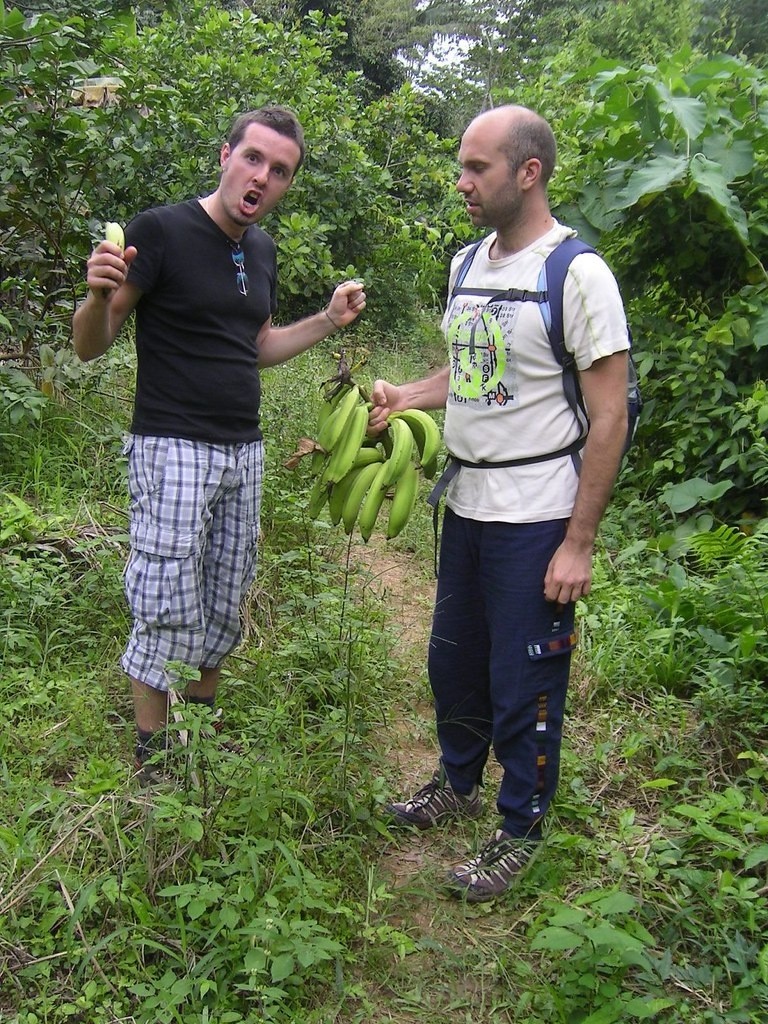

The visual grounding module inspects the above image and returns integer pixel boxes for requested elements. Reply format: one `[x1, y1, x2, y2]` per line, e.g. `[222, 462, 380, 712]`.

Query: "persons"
[368, 105, 632, 903]
[74, 107, 367, 784]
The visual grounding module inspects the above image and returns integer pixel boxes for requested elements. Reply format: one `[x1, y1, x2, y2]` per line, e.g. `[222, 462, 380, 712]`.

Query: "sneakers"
[384, 768, 484, 833]
[198, 707, 244, 757]
[134, 759, 182, 796]
[442, 825, 544, 905]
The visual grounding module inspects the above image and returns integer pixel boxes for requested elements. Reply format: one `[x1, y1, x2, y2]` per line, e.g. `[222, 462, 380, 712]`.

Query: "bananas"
[103, 220, 124, 299]
[310, 374, 442, 540]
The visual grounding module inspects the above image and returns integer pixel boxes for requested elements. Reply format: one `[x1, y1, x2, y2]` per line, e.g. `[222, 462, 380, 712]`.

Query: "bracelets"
[324, 309, 341, 329]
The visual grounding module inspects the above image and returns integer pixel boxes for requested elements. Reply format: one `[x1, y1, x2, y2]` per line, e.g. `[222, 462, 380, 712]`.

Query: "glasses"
[230, 244, 250, 296]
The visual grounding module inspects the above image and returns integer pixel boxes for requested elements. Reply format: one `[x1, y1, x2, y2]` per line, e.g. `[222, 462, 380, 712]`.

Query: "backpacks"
[449, 238, 642, 470]
